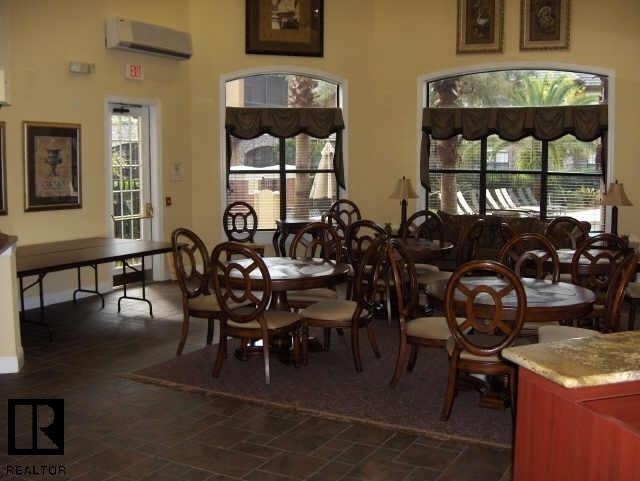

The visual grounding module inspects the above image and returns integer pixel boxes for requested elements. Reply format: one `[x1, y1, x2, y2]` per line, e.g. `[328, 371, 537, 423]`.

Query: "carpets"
[112, 312, 515, 451]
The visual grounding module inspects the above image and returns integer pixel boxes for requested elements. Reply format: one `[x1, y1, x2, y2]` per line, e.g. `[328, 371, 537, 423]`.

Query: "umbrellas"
[309, 141, 336, 200]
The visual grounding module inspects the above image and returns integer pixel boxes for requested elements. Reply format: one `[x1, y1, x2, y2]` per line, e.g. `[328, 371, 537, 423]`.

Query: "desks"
[16, 236, 189, 345]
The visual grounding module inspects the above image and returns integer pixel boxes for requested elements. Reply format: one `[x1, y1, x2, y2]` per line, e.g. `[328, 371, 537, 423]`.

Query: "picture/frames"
[245, 0, 324, 57]
[0, 121, 8, 215]
[22, 121, 83, 212]
[520, 0, 570, 49]
[456, 0, 504, 54]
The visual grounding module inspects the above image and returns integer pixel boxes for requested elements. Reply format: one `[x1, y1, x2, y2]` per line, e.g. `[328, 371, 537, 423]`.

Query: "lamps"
[387, 175, 420, 243]
[600, 179, 634, 247]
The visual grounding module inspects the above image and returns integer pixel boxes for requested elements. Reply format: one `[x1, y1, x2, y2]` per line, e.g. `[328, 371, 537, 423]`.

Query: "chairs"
[438, 188, 566, 216]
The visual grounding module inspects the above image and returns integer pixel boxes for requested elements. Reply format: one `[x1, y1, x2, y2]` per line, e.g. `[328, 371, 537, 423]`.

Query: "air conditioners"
[106, 16, 193, 61]
